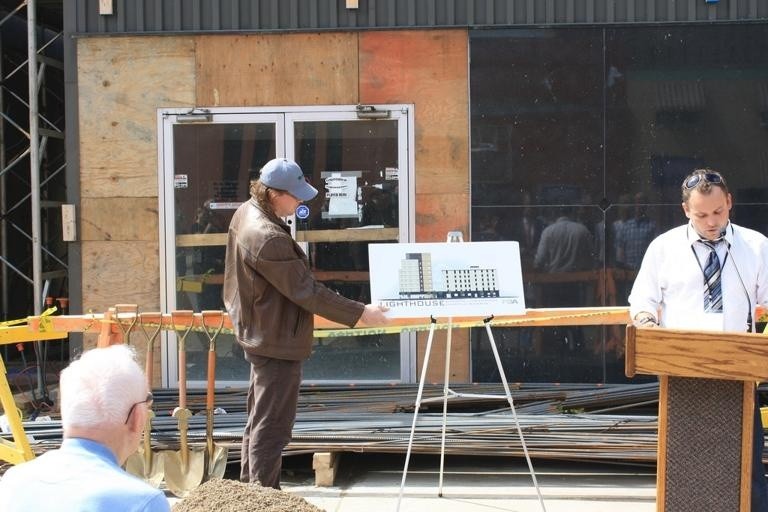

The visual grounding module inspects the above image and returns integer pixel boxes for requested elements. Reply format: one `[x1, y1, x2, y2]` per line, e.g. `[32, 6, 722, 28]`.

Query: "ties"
[698, 239, 724, 314]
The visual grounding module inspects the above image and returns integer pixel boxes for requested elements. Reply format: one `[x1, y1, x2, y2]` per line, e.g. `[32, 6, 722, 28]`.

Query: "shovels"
[112, 304, 229, 498]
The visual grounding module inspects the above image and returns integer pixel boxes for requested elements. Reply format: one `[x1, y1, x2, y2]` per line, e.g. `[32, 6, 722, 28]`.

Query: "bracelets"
[636, 316, 661, 325]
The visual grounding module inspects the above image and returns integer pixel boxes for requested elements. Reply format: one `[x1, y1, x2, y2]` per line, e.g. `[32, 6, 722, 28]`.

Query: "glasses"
[682, 173, 726, 190]
[124, 392, 153, 424]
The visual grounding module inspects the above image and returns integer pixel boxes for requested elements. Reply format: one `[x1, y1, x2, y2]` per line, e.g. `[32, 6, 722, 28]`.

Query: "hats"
[260, 157, 319, 201]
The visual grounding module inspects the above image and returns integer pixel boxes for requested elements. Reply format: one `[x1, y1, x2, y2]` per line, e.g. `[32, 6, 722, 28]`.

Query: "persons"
[222, 156, 392, 493]
[471, 188, 681, 369]
[626, 167, 768, 334]
[0, 343, 172, 511]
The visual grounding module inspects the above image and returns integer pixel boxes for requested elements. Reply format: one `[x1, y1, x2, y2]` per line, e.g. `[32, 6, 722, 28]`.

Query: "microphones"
[719, 227, 754, 333]
